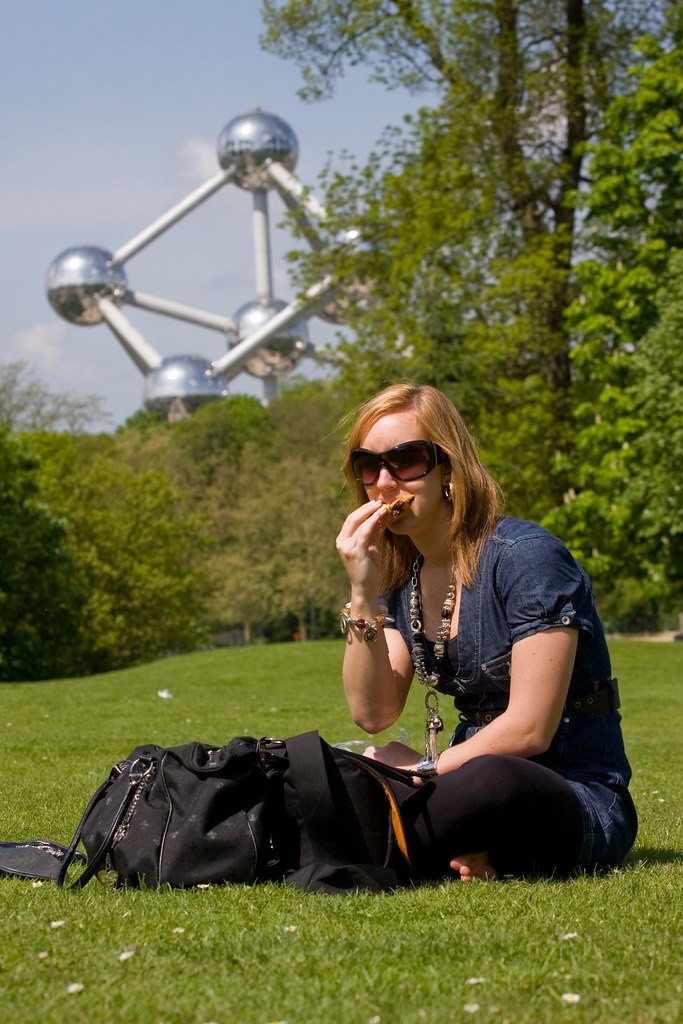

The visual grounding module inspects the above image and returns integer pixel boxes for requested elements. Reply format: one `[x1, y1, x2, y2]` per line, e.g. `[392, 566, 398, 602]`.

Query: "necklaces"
[410, 555, 457, 762]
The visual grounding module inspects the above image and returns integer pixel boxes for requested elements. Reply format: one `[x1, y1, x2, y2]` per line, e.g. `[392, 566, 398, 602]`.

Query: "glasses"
[351, 440, 448, 486]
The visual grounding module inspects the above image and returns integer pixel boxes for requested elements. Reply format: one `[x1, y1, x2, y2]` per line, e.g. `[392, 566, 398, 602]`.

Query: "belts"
[466, 678, 621, 731]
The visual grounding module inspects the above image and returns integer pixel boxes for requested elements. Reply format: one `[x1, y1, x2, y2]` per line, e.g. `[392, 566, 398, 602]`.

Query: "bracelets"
[338, 600, 388, 644]
[417, 750, 438, 782]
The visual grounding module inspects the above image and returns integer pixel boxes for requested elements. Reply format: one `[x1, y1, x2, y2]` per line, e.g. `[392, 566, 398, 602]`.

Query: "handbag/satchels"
[265, 730, 436, 892]
[59, 737, 414, 893]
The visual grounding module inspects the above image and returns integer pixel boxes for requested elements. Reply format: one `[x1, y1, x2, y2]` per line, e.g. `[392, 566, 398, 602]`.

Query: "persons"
[337, 382, 638, 893]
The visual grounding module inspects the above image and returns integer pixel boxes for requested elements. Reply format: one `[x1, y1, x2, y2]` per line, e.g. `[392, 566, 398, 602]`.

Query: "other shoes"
[0, 839, 88, 882]
